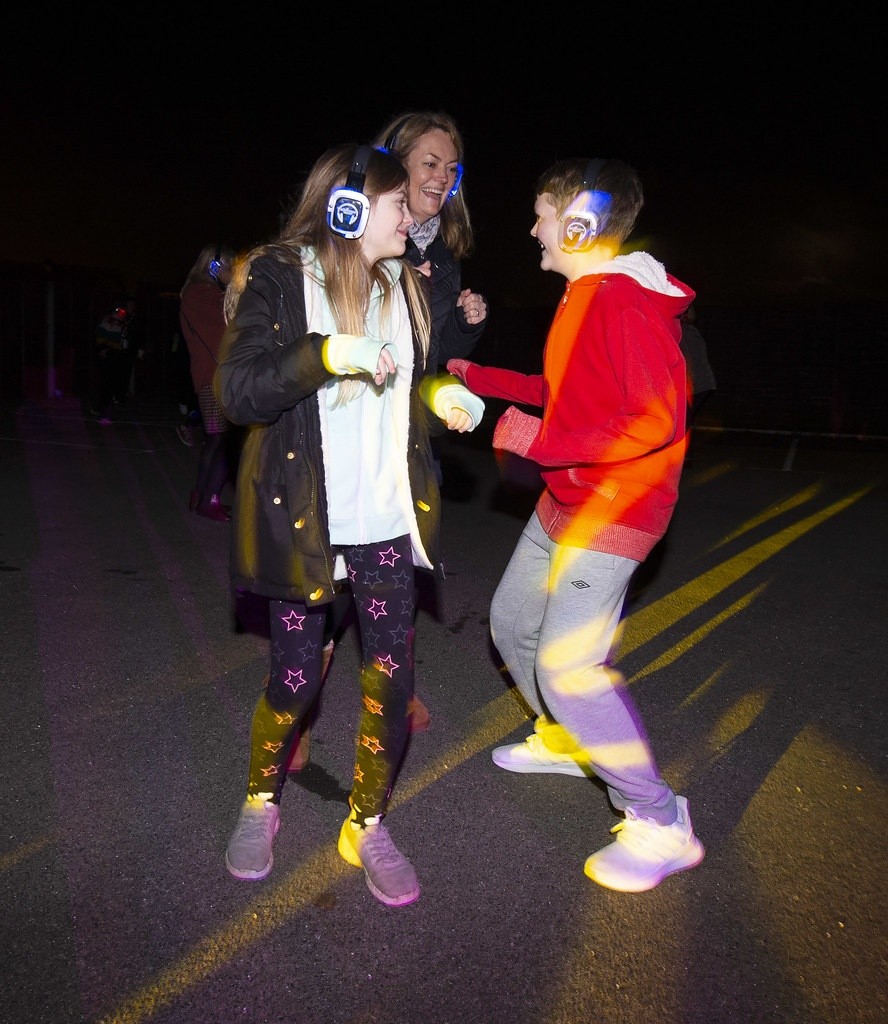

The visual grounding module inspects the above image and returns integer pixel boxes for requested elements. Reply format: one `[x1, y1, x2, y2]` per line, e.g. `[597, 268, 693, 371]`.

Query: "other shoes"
[189, 489, 233, 514]
[196, 492, 234, 523]
[177, 426, 193, 446]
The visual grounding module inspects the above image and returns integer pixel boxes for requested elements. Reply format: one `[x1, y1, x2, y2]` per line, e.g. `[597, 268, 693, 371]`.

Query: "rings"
[475, 309, 479, 317]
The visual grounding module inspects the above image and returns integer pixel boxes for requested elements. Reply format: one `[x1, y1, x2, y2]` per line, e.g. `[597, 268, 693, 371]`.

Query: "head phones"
[209, 246, 223, 278]
[326, 145, 376, 240]
[376, 116, 465, 198]
[557, 155, 610, 253]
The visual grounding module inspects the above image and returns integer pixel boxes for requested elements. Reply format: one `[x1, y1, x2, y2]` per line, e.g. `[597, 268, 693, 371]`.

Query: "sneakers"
[224, 803, 281, 881]
[337, 817, 420, 907]
[492, 733, 594, 777]
[583, 795, 706, 893]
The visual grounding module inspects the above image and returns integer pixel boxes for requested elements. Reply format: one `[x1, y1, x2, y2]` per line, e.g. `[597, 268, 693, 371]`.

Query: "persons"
[210, 145, 487, 906]
[285, 109, 495, 771]
[445, 146, 707, 895]
[87, 296, 146, 424]
[172, 226, 279, 520]
